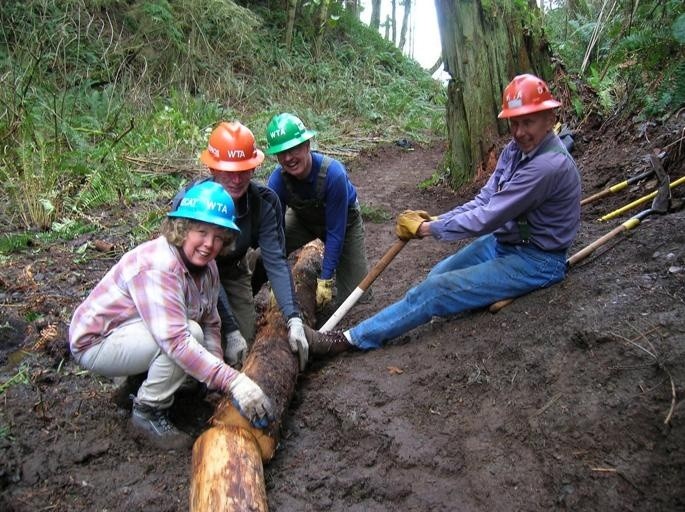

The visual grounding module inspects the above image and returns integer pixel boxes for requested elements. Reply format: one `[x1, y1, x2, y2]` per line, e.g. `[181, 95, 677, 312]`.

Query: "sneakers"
[129, 404, 193, 452]
[114, 379, 133, 406]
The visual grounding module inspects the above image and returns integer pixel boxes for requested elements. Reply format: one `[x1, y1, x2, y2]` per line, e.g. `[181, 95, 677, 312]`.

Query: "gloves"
[417, 208, 437, 221]
[224, 331, 247, 365]
[395, 208, 422, 240]
[227, 374, 278, 431]
[287, 317, 310, 371]
[315, 278, 338, 308]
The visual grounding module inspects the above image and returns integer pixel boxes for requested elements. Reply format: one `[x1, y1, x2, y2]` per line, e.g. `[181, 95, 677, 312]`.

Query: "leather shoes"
[305, 326, 350, 361]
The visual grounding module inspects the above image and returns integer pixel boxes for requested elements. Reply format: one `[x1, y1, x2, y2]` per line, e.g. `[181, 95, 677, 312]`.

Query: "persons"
[165, 125, 311, 373]
[68, 182, 279, 448]
[298, 74, 581, 356]
[252, 113, 372, 309]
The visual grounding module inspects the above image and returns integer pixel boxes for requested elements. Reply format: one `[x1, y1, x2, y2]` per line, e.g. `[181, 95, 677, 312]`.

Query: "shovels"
[579, 155, 671, 207]
[489, 175, 670, 313]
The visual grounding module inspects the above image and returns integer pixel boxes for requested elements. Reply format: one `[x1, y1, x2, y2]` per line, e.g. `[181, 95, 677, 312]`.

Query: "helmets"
[264, 112, 316, 154]
[168, 180, 240, 231]
[496, 74, 561, 119]
[200, 123, 264, 171]
